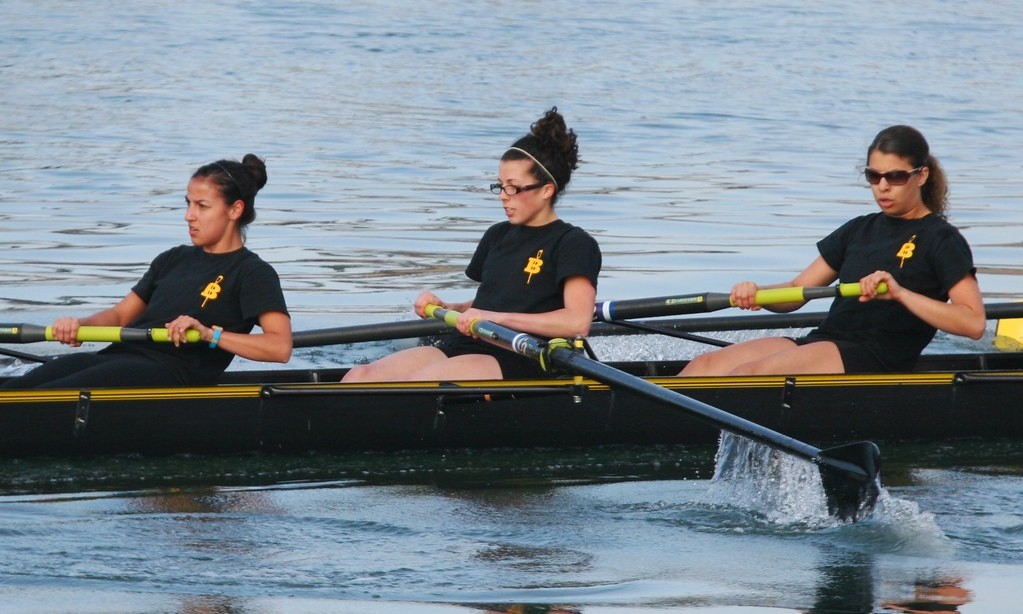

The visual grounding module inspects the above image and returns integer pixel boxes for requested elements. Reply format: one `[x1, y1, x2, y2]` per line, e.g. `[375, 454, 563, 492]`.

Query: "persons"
[3, 153, 298, 390]
[674, 121, 989, 371]
[337, 104, 606, 379]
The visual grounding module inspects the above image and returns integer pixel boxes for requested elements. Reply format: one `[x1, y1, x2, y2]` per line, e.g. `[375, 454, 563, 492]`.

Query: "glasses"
[490, 183, 546, 196]
[865, 165, 925, 185]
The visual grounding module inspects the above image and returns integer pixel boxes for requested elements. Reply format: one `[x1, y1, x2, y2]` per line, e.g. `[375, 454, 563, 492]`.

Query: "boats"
[1, 298, 1023, 457]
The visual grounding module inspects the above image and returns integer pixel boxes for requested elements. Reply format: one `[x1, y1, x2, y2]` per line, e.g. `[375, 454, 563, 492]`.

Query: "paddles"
[419, 302, 1019, 346]
[421, 303, 882, 527]
[0, 324, 204, 344]
[291, 280, 888, 348]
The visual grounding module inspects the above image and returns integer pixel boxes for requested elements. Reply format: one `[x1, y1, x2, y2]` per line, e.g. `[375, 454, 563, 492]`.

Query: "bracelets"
[206, 324, 224, 349]
